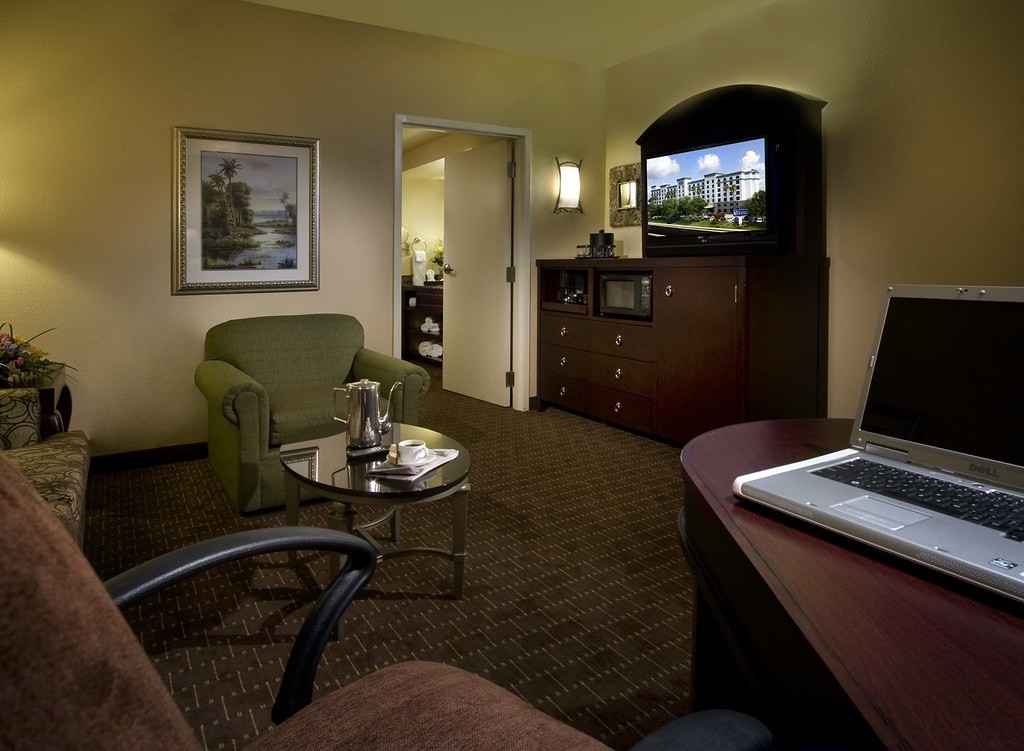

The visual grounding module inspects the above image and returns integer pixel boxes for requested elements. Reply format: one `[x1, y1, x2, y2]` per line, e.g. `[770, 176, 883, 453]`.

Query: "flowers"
[430, 245, 443, 267]
[0, 320, 78, 387]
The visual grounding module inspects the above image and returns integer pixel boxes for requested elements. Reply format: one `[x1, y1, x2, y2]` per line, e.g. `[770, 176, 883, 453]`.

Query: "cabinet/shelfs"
[536, 255, 828, 449]
[402, 275, 443, 368]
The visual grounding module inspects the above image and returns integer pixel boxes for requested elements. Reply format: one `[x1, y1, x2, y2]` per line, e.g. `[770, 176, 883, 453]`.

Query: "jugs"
[590, 229, 616, 257]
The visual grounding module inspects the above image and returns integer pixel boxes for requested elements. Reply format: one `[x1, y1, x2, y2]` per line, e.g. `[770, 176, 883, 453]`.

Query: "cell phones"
[346, 443, 399, 461]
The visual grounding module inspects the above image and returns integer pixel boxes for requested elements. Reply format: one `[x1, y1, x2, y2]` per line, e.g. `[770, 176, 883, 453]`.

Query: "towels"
[419, 341, 443, 358]
[411, 251, 426, 287]
[421, 317, 440, 332]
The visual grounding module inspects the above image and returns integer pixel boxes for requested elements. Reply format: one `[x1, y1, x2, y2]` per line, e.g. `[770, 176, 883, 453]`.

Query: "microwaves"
[597, 273, 653, 321]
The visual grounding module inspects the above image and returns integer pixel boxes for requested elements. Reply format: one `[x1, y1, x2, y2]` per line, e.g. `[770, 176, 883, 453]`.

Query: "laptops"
[730, 282, 1024, 603]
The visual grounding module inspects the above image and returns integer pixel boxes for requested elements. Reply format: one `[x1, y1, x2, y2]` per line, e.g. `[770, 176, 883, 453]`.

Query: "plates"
[386, 448, 436, 467]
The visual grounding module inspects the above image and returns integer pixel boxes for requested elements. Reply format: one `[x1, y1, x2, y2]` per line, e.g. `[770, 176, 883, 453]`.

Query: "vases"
[437, 267, 443, 280]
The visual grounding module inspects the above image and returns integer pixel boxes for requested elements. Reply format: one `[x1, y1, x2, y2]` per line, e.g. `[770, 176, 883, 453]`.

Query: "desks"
[278, 417, 474, 641]
[679, 420, 1024, 751]
[36, 364, 76, 440]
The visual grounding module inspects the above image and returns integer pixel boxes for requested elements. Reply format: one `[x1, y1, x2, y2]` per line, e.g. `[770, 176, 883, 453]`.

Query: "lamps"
[552, 157, 583, 214]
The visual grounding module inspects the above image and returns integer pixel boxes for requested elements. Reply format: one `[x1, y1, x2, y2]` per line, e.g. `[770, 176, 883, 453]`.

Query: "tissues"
[423, 269, 437, 286]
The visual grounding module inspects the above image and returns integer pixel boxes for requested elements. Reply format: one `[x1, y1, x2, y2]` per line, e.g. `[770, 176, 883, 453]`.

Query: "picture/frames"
[280, 447, 319, 481]
[172, 124, 320, 294]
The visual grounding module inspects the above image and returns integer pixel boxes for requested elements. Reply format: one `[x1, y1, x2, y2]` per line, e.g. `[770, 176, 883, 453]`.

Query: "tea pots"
[331, 379, 402, 449]
[332, 446, 382, 492]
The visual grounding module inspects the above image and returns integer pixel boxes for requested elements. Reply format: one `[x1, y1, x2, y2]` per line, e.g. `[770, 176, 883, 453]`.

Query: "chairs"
[0, 455, 772, 751]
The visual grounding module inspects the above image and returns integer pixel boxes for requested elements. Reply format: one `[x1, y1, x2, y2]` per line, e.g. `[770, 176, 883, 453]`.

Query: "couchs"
[193, 314, 430, 516]
[0, 388, 88, 554]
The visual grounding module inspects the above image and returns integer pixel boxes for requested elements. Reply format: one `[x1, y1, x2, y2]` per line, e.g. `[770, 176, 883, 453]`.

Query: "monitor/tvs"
[644, 130, 778, 248]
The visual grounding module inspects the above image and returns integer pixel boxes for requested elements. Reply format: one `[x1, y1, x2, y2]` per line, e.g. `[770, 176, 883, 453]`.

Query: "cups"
[576, 244, 616, 257]
[398, 439, 429, 462]
